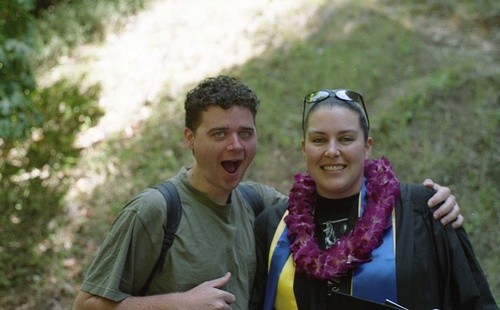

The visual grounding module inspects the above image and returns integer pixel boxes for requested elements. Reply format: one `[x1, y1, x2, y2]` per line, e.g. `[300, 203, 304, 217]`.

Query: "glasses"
[301, 90, 369, 130]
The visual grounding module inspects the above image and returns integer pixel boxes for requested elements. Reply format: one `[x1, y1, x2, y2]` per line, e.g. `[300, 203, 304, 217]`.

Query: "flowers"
[284, 156, 400, 279]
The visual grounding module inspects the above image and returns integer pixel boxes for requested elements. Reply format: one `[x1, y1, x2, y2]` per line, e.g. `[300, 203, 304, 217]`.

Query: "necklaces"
[284, 155, 400, 282]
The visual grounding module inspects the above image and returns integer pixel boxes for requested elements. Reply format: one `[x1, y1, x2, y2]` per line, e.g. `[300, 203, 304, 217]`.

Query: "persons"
[249, 89, 499, 310]
[71, 76, 464, 310]
